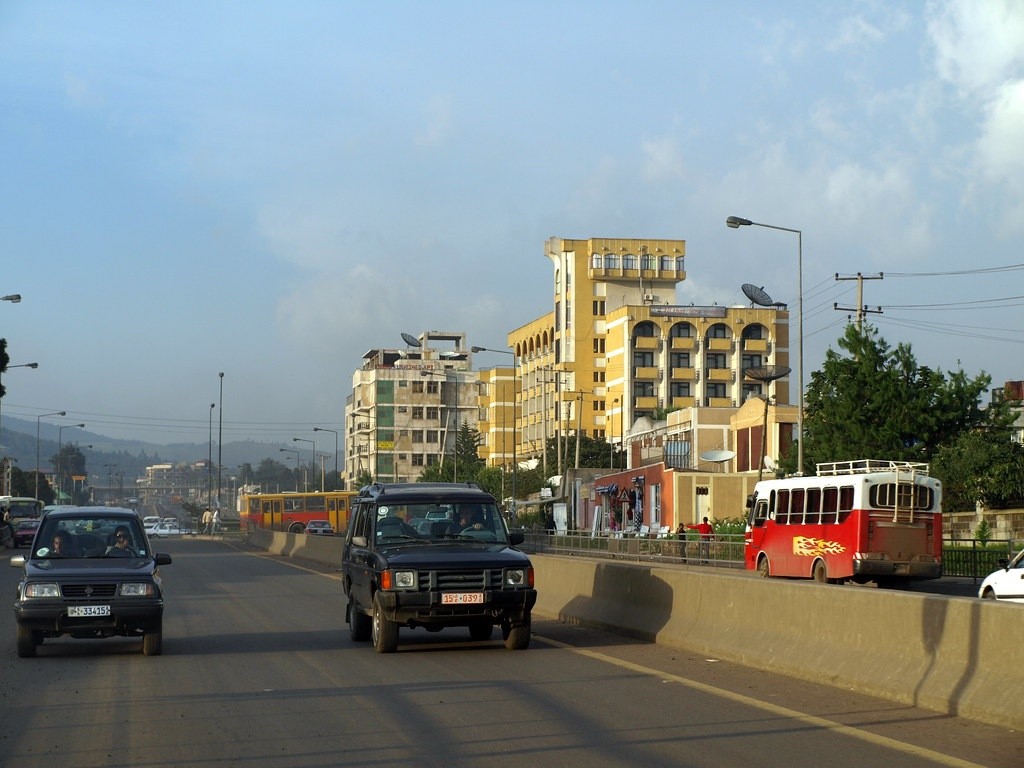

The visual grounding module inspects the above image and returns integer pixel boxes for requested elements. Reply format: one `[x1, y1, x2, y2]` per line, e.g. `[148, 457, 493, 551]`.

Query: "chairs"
[431, 522, 452, 536]
[79, 534, 106, 557]
[64, 535, 83, 558]
[110, 534, 134, 547]
[403, 522, 420, 536]
[444, 513, 482, 540]
[376, 517, 408, 536]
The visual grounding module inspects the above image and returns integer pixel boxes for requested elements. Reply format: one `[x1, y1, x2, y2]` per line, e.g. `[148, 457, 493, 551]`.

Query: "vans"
[43, 506, 80, 532]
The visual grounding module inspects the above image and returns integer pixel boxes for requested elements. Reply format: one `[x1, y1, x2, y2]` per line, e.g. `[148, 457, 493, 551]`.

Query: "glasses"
[115, 533, 130, 538]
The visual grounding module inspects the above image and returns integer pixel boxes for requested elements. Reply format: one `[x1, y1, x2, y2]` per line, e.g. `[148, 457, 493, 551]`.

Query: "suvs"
[11, 507, 173, 654]
[341, 482, 538, 650]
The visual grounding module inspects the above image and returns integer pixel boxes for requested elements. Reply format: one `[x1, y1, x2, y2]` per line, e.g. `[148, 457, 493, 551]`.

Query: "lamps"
[712, 301, 718, 306]
[689, 301, 694, 306]
[664, 301, 669, 305]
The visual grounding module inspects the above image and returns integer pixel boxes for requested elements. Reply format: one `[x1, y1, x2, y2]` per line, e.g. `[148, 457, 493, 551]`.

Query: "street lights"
[294, 438, 315, 488]
[208, 371, 225, 511]
[726, 218, 802, 473]
[279, 448, 299, 487]
[421, 371, 458, 482]
[35, 411, 92, 500]
[314, 428, 338, 488]
[350, 412, 377, 484]
[472, 346, 517, 531]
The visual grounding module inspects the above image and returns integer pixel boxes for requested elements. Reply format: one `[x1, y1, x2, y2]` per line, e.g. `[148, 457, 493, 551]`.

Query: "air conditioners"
[643, 294, 654, 301]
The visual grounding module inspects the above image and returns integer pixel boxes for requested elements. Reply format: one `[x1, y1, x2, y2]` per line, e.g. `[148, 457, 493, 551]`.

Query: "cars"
[15, 520, 40, 546]
[99, 496, 166, 506]
[304, 521, 334, 535]
[977, 547, 1024, 606]
[73, 517, 200, 538]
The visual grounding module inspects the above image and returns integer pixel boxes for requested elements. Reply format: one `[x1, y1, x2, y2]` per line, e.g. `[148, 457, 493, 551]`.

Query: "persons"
[675, 522, 687, 565]
[210, 508, 223, 535]
[686, 517, 716, 566]
[2, 507, 21, 548]
[104, 526, 134, 555]
[544, 514, 557, 547]
[442, 505, 487, 541]
[52, 531, 75, 558]
[202, 507, 213, 535]
[260, 497, 348, 517]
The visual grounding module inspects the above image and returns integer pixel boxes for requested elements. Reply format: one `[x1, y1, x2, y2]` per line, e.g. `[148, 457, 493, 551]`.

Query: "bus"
[744, 458, 945, 584]
[239, 491, 409, 533]
[7, 498, 45, 544]
